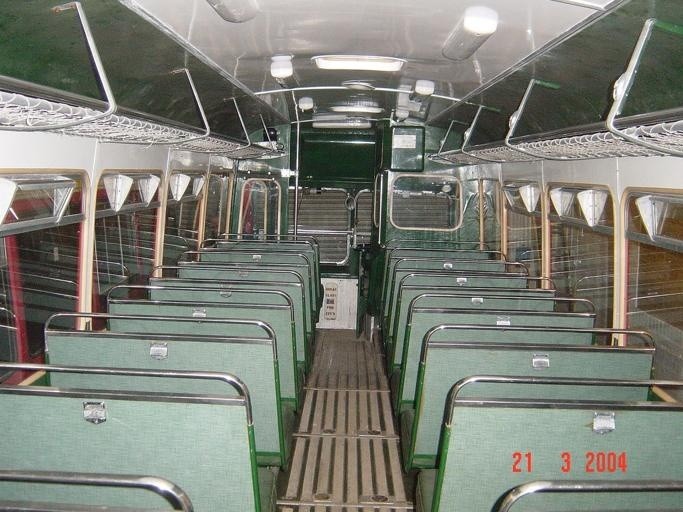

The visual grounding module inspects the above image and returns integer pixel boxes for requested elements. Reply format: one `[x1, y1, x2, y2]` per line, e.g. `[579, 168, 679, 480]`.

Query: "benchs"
[0, 233, 322, 512]
[382, 236, 683, 510]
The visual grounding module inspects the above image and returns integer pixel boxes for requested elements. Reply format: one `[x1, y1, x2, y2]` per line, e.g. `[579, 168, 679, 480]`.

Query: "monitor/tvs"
[298, 133, 378, 183]
